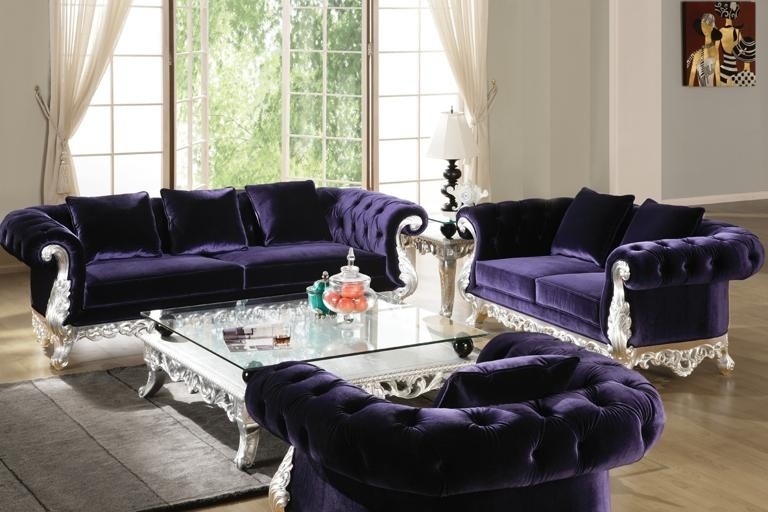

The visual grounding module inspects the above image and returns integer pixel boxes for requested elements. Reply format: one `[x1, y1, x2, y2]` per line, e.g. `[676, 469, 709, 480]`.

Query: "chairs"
[243, 329, 666, 512]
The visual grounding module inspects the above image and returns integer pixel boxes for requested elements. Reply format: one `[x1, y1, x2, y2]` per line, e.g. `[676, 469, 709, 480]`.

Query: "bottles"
[322, 246, 377, 324]
[305, 271, 339, 316]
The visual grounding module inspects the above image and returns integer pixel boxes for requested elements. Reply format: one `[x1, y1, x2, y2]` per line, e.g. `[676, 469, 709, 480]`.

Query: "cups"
[272, 322, 292, 350]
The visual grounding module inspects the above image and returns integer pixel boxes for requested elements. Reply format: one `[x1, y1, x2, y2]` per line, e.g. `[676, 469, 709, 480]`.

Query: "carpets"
[0, 363, 284, 509]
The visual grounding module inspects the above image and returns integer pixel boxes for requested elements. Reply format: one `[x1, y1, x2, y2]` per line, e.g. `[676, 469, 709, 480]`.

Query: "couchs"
[456, 196, 767, 375]
[0, 187, 428, 376]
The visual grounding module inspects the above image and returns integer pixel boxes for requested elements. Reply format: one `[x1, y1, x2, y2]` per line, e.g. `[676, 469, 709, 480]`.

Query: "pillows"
[160, 188, 249, 256]
[548, 187, 635, 268]
[245, 180, 333, 246]
[620, 199, 706, 245]
[63, 192, 163, 265]
[437, 353, 580, 406]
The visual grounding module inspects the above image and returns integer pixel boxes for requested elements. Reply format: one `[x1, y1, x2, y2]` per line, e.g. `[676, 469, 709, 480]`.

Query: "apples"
[323, 282, 368, 314]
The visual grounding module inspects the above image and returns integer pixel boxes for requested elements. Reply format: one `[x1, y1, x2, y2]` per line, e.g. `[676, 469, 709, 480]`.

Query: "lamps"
[428, 104, 479, 214]
